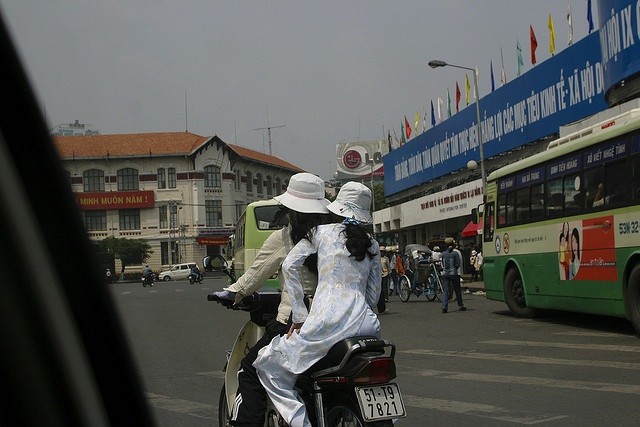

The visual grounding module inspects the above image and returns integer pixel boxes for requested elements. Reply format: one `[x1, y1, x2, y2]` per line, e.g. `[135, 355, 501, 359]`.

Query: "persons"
[214, 172, 333, 427]
[569, 228, 581, 279]
[559, 233, 568, 279]
[252, 181, 384, 426]
[391, 251, 397, 294]
[441, 238, 467, 313]
[563, 223, 571, 264]
[396, 250, 404, 276]
[477, 252, 483, 277]
[448, 243, 464, 302]
[470, 250, 479, 283]
[594, 184, 609, 207]
[142, 264, 152, 282]
[428, 246, 442, 289]
[380, 247, 390, 302]
[190, 265, 199, 281]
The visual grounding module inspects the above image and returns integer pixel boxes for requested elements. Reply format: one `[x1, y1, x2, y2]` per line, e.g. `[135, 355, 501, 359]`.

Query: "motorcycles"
[187, 271, 203, 285]
[203, 255, 407, 427]
[141, 273, 155, 287]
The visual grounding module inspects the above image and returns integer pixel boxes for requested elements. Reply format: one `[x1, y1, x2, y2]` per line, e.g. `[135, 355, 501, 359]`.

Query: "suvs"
[158, 263, 200, 281]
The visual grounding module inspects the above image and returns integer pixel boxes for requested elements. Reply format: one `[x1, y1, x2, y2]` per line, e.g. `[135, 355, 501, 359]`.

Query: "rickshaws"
[397, 244, 450, 303]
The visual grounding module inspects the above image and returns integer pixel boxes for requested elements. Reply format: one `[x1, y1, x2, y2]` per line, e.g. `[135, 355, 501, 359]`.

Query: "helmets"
[145, 264, 148, 267]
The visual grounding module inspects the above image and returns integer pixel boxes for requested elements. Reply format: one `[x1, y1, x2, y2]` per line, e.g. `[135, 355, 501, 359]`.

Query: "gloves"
[213, 288, 236, 301]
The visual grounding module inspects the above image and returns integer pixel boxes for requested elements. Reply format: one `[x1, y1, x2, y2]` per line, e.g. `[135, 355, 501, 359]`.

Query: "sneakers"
[441, 306, 447, 313]
[459, 307, 467, 311]
[379, 309, 390, 315]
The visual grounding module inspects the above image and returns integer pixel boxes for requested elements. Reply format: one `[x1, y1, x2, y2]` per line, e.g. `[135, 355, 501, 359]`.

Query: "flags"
[528, 24, 539, 65]
[415, 113, 420, 135]
[516, 39, 524, 74]
[388, 130, 393, 152]
[491, 61, 495, 92]
[474, 67, 479, 99]
[393, 131, 400, 149]
[422, 111, 426, 131]
[501, 50, 506, 85]
[447, 90, 451, 118]
[566, 1, 574, 46]
[437, 97, 444, 123]
[404, 115, 411, 138]
[400, 123, 406, 145]
[465, 74, 471, 104]
[547, 12, 556, 57]
[587, 1, 594, 34]
[455, 81, 461, 111]
[431, 100, 436, 126]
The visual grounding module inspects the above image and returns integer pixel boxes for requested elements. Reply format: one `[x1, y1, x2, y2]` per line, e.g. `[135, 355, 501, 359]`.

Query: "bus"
[223, 200, 289, 294]
[472, 109, 640, 336]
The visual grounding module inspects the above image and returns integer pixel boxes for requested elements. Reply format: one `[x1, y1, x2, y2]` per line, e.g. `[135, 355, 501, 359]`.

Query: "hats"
[273, 173, 331, 214]
[472, 250, 477, 255]
[380, 246, 386, 251]
[445, 238, 455, 243]
[326, 181, 374, 224]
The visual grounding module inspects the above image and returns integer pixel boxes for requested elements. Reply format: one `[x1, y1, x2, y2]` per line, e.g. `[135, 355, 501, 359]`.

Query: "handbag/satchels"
[469, 265, 476, 271]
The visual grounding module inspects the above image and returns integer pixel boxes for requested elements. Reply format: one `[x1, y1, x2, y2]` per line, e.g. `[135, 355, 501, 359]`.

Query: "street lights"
[348, 159, 375, 237]
[428, 60, 486, 196]
